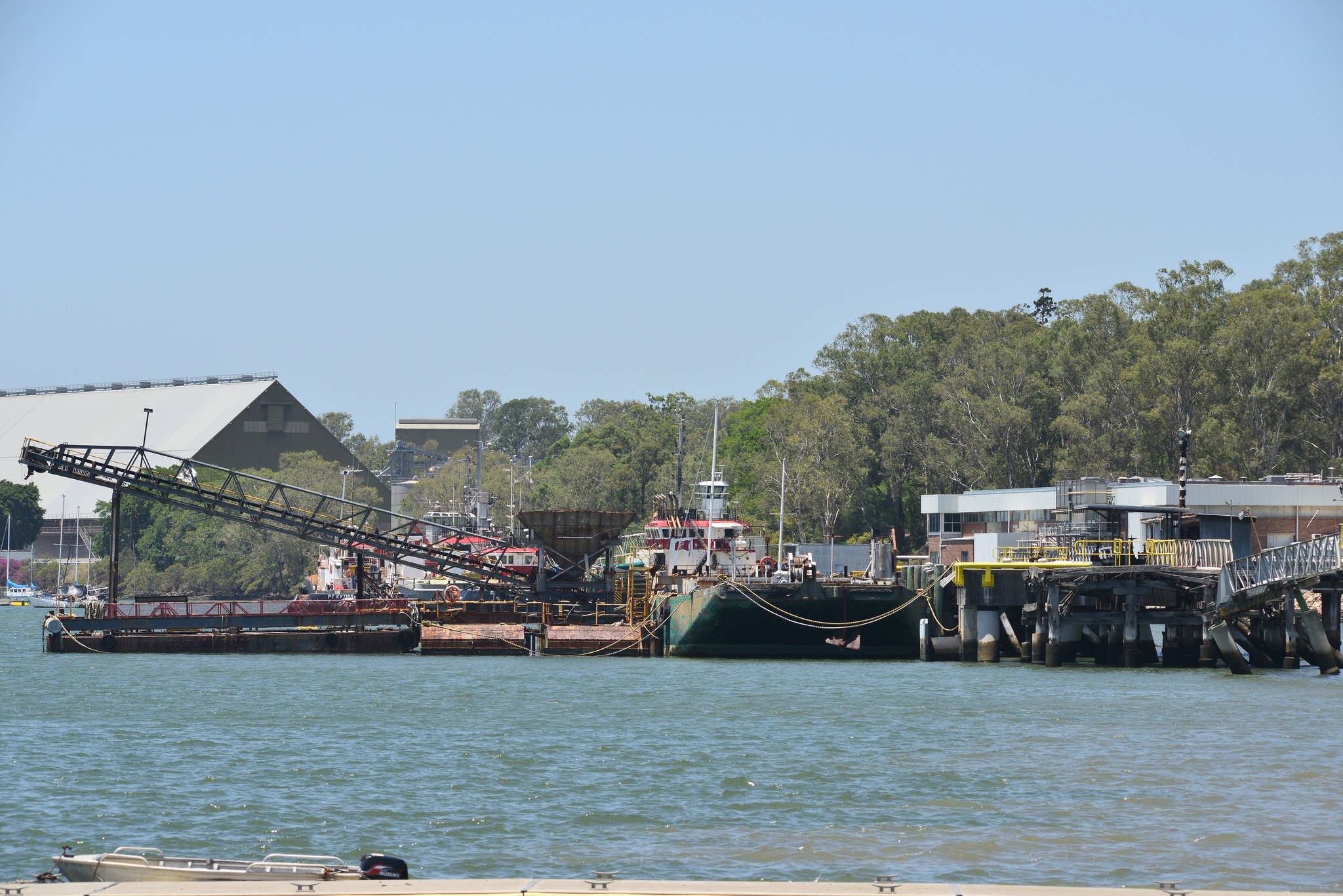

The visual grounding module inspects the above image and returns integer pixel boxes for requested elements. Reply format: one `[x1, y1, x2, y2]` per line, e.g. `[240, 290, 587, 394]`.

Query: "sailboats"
[0, 493, 110, 609]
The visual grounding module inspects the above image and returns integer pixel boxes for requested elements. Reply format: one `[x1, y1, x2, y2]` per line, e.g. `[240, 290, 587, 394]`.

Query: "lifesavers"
[444, 585, 461, 602]
[760, 556, 774, 570]
[658, 601, 671, 625]
[397, 628, 414, 646]
[101, 634, 116, 649]
[324, 633, 338, 646]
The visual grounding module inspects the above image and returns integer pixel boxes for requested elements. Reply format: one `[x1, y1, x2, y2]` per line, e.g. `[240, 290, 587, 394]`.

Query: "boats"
[50, 846, 409, 882]
[284, 397, 818, 613]
